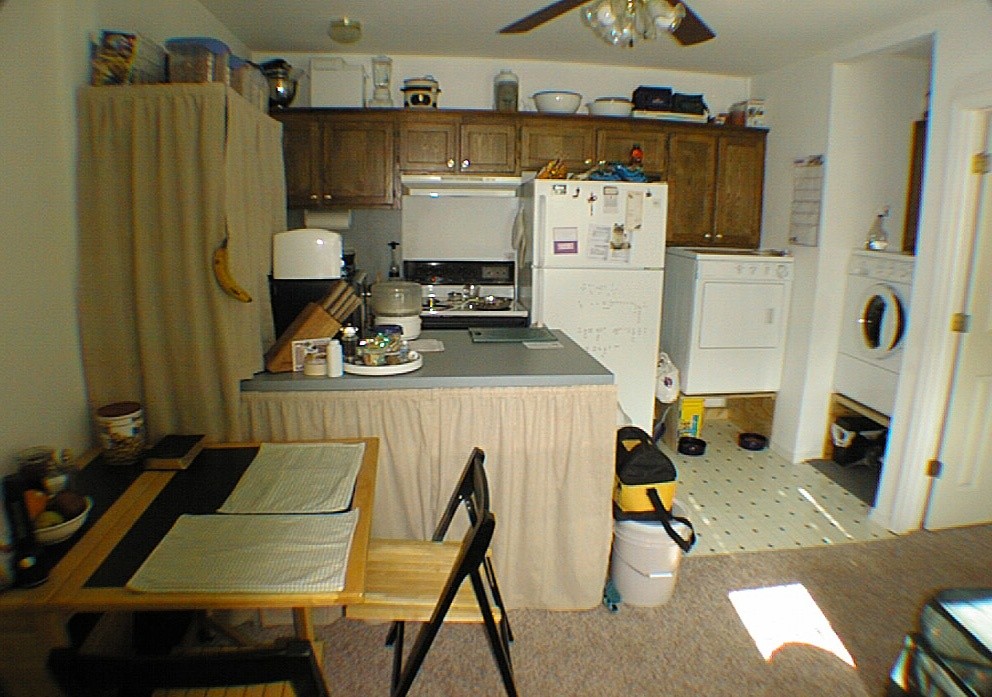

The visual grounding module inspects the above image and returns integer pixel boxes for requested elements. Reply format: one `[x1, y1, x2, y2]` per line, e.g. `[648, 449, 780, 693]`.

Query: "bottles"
[389, 246, 399, 280]
[326, 339, 343, 378]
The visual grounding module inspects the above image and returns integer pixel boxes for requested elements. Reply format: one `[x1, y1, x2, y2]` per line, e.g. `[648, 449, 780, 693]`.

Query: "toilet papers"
[303, 209, 352, 229]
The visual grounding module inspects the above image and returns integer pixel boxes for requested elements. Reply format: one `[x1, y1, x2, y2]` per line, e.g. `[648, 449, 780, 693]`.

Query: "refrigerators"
[515, 177, 671, 438]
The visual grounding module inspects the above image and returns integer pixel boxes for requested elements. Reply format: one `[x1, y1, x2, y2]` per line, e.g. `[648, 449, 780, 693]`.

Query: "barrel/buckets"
[612, 500, 690, 610]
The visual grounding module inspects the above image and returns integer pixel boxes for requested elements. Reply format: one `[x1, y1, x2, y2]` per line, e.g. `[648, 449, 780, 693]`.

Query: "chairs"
[46, 638, 330, 697]
[347, 446, 520, 697]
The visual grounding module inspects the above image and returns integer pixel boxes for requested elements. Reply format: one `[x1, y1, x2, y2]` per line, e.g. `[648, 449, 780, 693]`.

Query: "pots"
[260, 59, 299, 110]
[461, 295, 512, 310]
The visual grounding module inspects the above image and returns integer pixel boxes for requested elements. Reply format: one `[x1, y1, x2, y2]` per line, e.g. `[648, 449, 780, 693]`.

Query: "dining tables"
[0, 435, 379, 665]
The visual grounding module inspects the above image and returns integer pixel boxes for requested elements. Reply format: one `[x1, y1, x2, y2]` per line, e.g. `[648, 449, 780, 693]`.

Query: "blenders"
[366, 55, 394, 107]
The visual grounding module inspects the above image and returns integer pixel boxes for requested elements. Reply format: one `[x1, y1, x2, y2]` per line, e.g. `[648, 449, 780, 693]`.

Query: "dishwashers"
[665, 244, 794, 395]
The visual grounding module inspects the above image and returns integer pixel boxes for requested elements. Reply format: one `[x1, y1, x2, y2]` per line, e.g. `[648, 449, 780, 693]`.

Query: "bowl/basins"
[29, 494, 92, 544]
[534, 92, 582, 113]
[585, 98, 632, 117]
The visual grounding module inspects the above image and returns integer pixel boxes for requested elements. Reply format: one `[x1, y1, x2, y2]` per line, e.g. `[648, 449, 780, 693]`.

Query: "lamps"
[328, 18, 362, 44]
[497, 0, 716, 50]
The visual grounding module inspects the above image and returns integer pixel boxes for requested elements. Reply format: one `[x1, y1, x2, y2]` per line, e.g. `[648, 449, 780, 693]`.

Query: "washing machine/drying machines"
[834, 246, 920, 419]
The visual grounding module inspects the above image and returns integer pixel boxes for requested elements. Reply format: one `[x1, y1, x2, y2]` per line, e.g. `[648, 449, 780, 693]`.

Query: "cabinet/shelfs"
[518, 111, 667, 174]
[398, 108, 522, 183]
[272, 108, 399, 210]
[666, 120, 770, 248]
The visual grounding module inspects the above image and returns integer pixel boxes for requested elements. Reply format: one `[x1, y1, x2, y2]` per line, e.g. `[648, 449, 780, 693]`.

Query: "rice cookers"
[401, 76, 440, 108]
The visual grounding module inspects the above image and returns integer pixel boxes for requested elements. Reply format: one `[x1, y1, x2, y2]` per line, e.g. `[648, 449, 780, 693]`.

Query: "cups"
[93, 400, 146, 466]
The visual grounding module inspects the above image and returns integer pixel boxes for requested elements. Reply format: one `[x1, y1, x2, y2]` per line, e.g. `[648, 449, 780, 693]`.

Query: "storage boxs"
[164, 37, 232, 85]
[91, 29, 168, 86]
[231, 54, 270, 114]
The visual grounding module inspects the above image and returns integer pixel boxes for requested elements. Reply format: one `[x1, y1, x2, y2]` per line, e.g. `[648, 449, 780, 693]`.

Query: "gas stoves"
[403, 262, 517, 313]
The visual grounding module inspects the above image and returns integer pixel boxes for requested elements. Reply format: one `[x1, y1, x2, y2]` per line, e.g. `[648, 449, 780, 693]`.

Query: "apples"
[25, 485, 88, 528]
